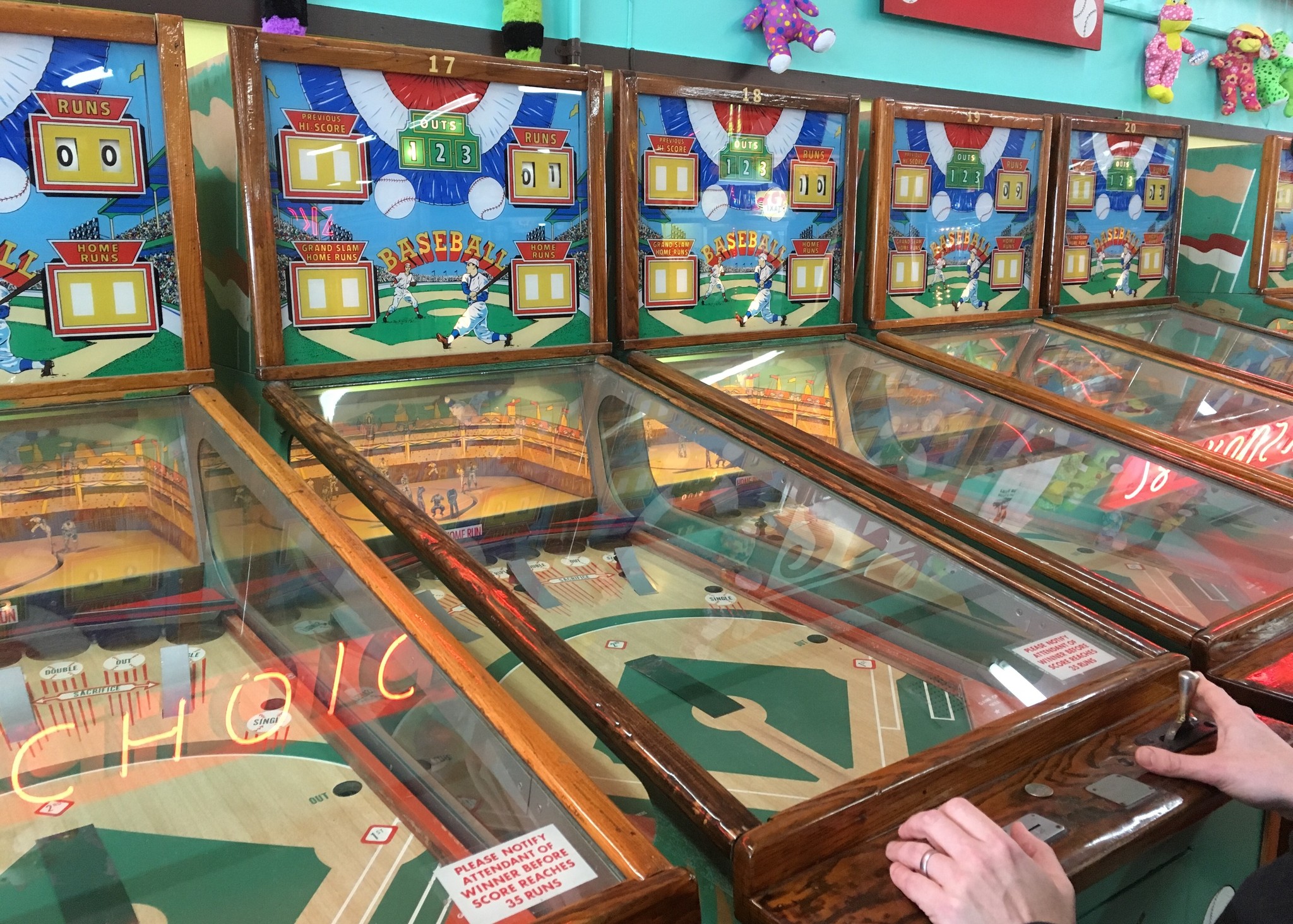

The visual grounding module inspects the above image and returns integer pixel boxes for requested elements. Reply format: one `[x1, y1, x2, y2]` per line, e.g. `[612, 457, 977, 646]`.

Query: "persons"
[885, 671, 1293, 924]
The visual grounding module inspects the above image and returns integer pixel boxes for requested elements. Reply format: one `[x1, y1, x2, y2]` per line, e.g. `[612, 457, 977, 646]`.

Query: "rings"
[920, 849, 939, 877]
